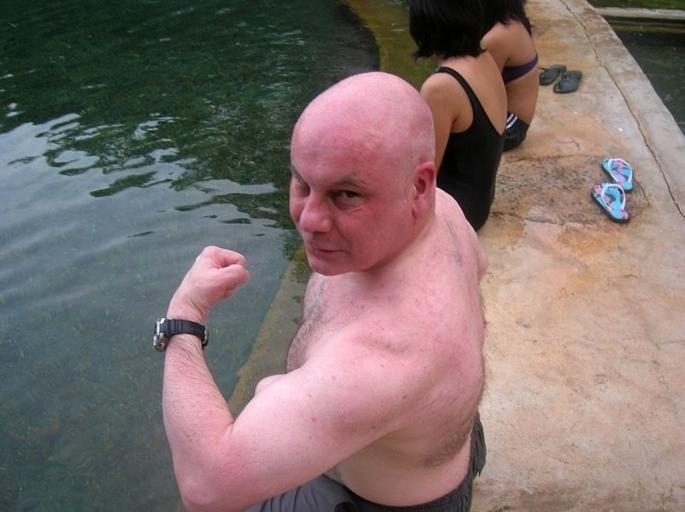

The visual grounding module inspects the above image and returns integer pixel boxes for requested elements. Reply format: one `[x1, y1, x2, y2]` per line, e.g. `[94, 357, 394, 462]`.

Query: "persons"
[480, 0, 540, 152]
[407, 1, 507, 232]
[153, 72, 491, 512]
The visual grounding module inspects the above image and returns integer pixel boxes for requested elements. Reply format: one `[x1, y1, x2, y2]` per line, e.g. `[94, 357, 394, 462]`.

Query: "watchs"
[153, 317, 210, 353]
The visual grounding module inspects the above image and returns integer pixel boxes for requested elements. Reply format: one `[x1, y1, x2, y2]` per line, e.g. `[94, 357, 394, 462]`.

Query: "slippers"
[591, 183, 631, 223]
[553, 70, 581, 93]
[538, 64, 567, 86]
[601, 158, 633, 192]
